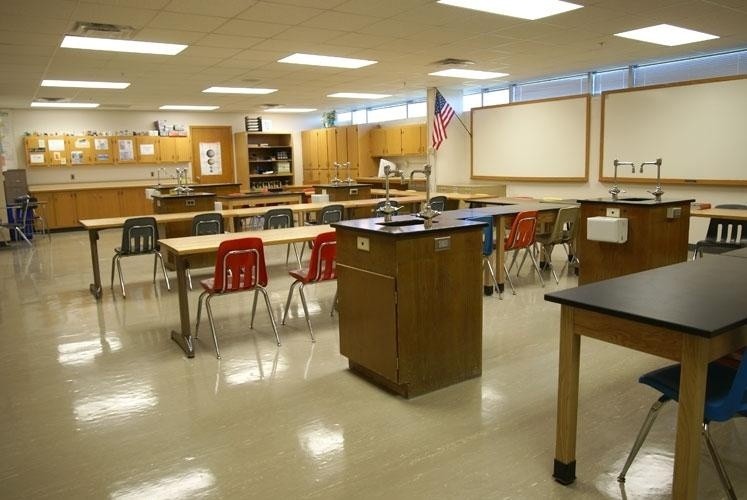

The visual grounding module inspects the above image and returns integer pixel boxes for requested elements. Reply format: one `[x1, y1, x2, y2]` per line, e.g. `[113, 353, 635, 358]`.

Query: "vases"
[323, 119, 334, 128]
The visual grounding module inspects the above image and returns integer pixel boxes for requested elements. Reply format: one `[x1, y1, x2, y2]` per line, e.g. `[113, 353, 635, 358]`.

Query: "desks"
[546, 244, 746, 500]
[216, 191, 304, 233]
[77, 190, 498, 300]
[688, 207, 746, 226]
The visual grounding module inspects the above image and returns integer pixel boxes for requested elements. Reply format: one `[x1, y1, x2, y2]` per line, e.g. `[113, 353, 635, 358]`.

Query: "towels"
[378, 159, 397, 177]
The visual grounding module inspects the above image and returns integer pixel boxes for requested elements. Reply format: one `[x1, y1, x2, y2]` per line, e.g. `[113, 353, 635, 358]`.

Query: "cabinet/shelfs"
[370, 124, 402, 158]
[26, 185, 155, 234]
[234, 130, 295, 192]
[402, 122, 428, 157]
[302, 124, 380, 183]
[22, 134, 193, 167]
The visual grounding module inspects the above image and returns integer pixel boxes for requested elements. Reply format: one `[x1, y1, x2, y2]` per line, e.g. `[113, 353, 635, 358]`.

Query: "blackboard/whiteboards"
[599, 75, 747, 185]
[471, 94, 590, 182]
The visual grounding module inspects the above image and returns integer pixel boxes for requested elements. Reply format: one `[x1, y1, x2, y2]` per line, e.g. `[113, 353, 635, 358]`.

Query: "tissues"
[311, 190, 329, 204]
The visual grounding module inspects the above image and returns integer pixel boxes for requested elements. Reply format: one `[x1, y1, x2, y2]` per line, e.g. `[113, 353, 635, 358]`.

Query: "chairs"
[615, 345, 746, 500]
[692, 203, 746, 260]
[0, 192, 49, 247]
[110, 195, 582, 363]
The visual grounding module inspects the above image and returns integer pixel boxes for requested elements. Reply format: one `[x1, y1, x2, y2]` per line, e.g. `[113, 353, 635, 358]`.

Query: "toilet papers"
[214, 202, 222, 211]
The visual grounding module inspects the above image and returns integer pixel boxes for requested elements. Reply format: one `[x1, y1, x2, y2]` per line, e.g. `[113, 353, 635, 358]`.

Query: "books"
[693, 203, 712, 210]
[248, 142, 292, 191]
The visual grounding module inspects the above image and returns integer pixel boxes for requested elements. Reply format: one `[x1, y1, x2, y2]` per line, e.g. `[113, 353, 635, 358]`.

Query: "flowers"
[319, 110, 338, 122]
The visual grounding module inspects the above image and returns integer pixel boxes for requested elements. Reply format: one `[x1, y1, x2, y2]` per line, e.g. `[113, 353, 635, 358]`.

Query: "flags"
[429, 86, 455, 153]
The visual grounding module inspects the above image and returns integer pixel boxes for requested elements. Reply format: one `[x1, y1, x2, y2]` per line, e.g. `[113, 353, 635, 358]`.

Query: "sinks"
[375, 219, 438, 228]
[616, 198, 655, 202]
[173, 193, 191, 196]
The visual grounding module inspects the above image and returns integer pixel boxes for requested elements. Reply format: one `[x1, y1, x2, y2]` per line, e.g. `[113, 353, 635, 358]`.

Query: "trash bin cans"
[6, 203, 33, 241]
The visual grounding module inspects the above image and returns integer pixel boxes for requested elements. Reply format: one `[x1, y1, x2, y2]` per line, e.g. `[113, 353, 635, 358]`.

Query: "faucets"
[343, 162, 351, 184]
[640, 158, 664, 201]
[157, 167, 168, 185]
[610, 160, 635, 194]
[332, 163, 341, 184]
[176, 168, 194, 194]
[383, 165, 405, 221]
[409, 164, 441, 227]
